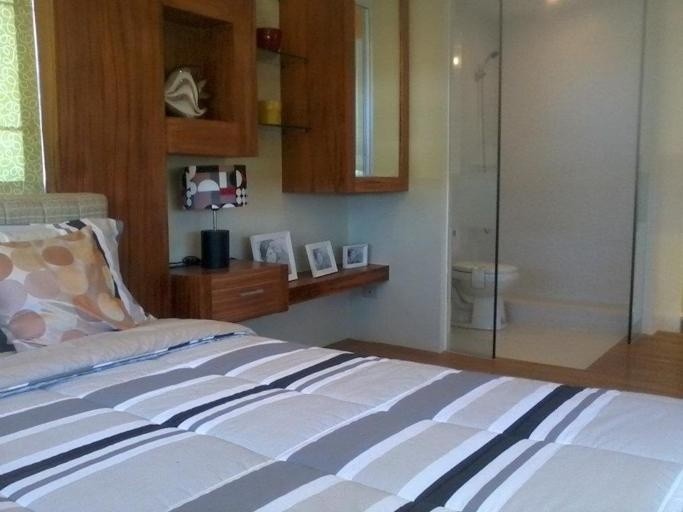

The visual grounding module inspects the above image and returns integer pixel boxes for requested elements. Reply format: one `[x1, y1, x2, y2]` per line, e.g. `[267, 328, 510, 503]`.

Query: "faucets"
[475, 51, 498, 80]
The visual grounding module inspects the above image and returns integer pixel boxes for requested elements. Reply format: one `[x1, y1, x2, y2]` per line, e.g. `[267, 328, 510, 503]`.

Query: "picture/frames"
[250, 230, 369, 282]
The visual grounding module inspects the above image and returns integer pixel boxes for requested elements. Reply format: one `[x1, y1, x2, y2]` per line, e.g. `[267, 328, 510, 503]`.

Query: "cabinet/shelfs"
[167, 261, 288, 321]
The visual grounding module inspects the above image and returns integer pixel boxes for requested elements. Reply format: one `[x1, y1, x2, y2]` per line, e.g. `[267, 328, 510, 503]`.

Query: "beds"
[0, 193, 682, 510]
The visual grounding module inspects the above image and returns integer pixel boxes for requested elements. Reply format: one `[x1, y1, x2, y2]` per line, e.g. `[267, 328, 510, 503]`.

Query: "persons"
[313, 247, 324, 269]
[261, 238, 282, 264]
[347, 248, 359, 264]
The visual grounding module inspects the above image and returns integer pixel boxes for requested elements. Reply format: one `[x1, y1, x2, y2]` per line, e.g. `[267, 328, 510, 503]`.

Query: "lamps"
[178, 165, 248, 269]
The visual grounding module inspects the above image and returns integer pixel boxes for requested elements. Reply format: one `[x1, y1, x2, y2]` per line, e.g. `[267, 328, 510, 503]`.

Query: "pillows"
[0, 216, 161, 352]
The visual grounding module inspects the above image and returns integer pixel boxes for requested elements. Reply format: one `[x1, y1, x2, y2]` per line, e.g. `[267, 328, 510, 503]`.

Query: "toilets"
[450, 262, 518, 330]
